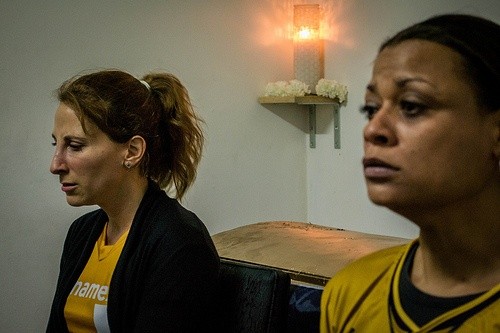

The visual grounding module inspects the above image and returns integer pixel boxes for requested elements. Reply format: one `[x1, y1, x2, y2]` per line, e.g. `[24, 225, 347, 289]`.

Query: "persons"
[319, 14, 500, 333]
[45, 69, 221, 333]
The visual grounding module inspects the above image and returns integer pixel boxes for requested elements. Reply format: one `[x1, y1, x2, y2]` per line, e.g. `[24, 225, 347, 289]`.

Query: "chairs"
[219, 260, 291, 333]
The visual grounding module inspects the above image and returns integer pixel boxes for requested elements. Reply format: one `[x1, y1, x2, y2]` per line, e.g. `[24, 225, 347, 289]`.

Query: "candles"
[292, 28, 325, 95]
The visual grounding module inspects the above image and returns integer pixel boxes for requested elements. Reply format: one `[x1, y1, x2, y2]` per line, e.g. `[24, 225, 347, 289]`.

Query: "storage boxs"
[210, 221, 413, 333]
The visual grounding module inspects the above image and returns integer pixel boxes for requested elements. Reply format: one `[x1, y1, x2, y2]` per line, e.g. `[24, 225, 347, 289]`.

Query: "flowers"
[265, 79, 348, 102]
